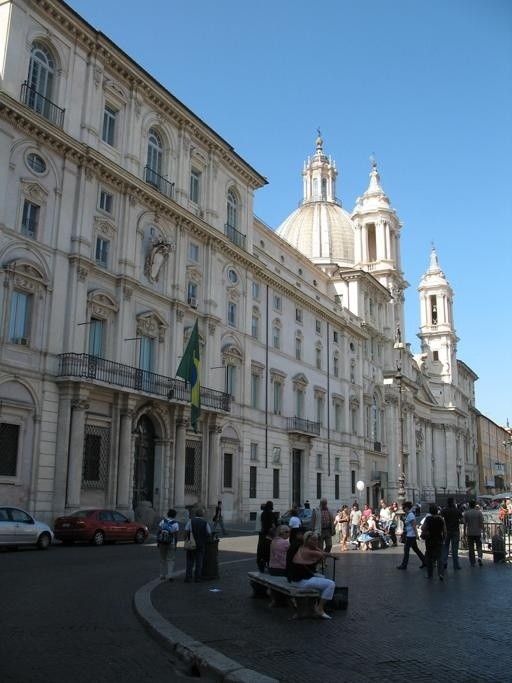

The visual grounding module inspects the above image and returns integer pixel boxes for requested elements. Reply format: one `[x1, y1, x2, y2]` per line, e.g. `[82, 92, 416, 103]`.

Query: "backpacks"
[157, 520, 173, 544]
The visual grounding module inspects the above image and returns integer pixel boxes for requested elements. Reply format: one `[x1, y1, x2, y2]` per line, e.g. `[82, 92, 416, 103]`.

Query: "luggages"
[321, 557, 348, 611]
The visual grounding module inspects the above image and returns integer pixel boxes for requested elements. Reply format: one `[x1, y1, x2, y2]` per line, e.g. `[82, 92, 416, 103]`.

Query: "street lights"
[393, 324, 410, 536]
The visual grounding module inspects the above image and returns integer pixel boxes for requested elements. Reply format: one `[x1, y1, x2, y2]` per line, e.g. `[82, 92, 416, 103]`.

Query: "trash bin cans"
[203, 531, 220, 579]
[492, 536, 506, 562]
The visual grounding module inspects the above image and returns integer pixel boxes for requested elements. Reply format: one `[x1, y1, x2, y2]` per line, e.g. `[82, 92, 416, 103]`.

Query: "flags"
[177, 317, 201, 436]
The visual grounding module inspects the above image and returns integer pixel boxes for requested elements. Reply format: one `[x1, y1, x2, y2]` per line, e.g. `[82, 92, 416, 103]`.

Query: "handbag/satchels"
[184, 533, 197, 550]
[420, 529, 431, 539]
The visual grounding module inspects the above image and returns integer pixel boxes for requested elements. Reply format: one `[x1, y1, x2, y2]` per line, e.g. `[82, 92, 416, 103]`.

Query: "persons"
[156, 508, 178, 581]
[245, 485, 512, 619]
[213, 499, 230, 537]
[184, 506, 212, 582]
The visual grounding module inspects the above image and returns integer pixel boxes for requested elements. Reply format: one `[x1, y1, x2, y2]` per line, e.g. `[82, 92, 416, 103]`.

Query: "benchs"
[358, 530, 391, 551]
[248, 569, 324, 622]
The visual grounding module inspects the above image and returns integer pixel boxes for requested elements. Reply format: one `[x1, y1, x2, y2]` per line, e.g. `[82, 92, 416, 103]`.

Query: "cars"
[54, 508, 149, 545]
[0, 506, 54, 548]
